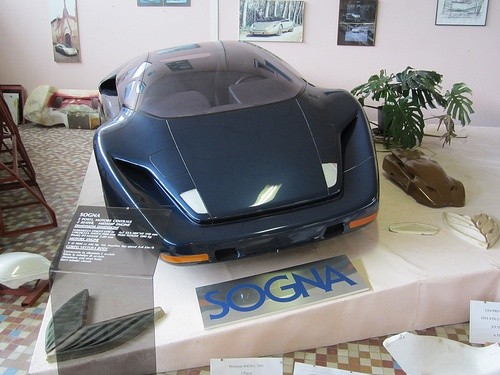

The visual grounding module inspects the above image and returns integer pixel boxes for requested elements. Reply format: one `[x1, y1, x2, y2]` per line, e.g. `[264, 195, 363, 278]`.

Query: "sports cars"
[382, 148, 466, 208]
[91, 38, 381, 266]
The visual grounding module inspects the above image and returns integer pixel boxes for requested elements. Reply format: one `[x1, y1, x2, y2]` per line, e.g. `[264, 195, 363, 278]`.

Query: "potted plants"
[350, 66, 474, 150]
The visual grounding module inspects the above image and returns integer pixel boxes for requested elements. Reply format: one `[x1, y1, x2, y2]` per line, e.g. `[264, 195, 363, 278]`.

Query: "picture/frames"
[435, 0, 489, 26]
[136, 0, 190, 6]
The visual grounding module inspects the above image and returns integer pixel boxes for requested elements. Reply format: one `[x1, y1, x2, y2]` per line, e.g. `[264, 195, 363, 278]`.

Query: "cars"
[247, 16, 294, 37]
[55, 44, 78, 56]
